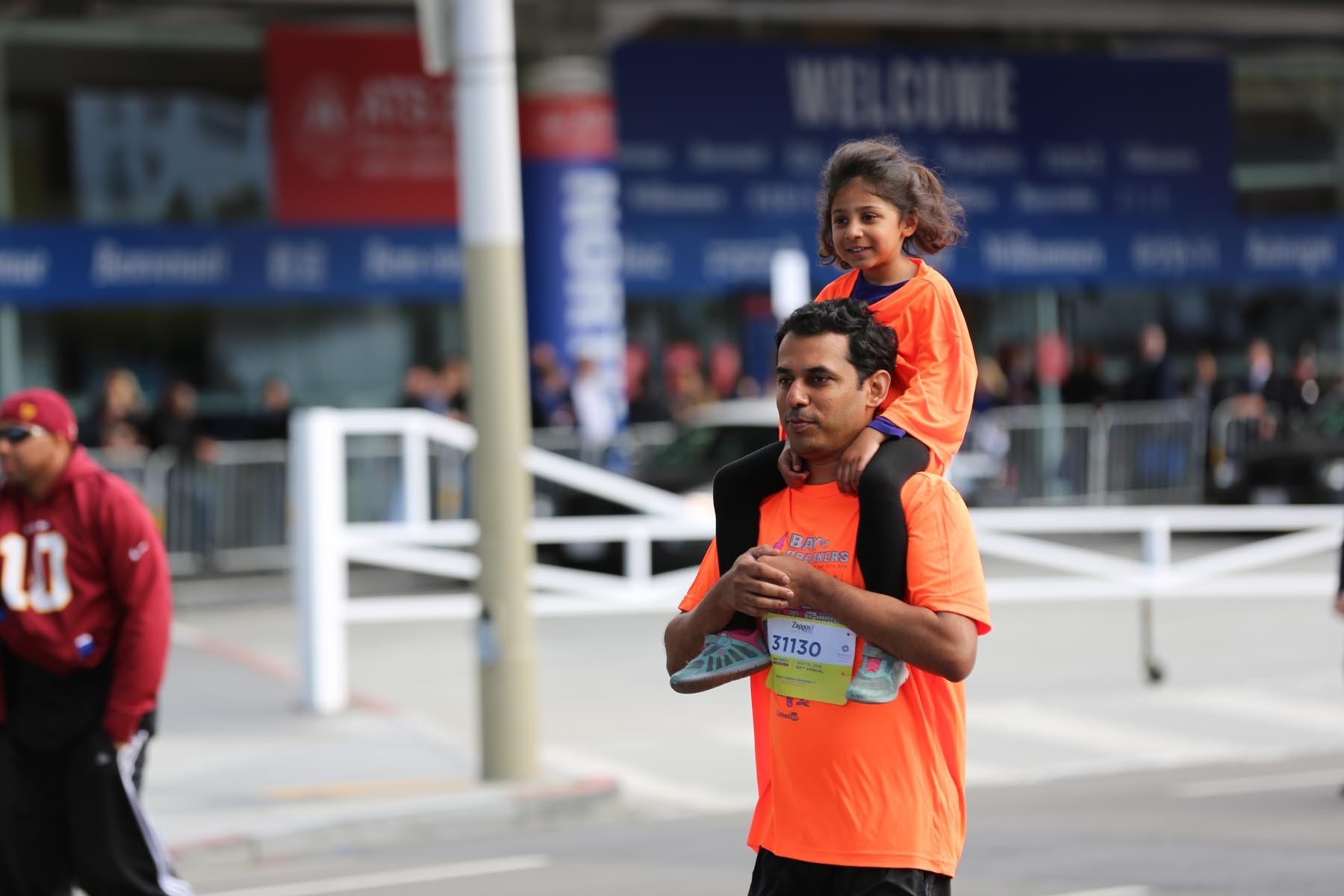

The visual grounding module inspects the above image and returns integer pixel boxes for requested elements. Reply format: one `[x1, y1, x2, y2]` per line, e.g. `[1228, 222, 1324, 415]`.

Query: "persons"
[670, 139, 980, 704]
[79, 363, 296, 460]
[401, 352, 474, 430]
[660, 299, 991, 896]
[1, 386, 206, 896]
[532, 281, 1341, 625]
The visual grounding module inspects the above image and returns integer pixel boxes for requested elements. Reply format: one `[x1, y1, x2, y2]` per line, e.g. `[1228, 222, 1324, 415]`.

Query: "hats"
[0, 387, 78, 448]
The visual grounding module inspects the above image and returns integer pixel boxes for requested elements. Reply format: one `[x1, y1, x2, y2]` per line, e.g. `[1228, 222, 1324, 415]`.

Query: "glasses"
[0, 427, 56, 443]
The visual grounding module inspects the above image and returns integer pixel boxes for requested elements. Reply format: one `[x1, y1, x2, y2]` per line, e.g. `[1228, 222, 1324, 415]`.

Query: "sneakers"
[846, 641, 910, 704]
[670, 627, 772, 694]
